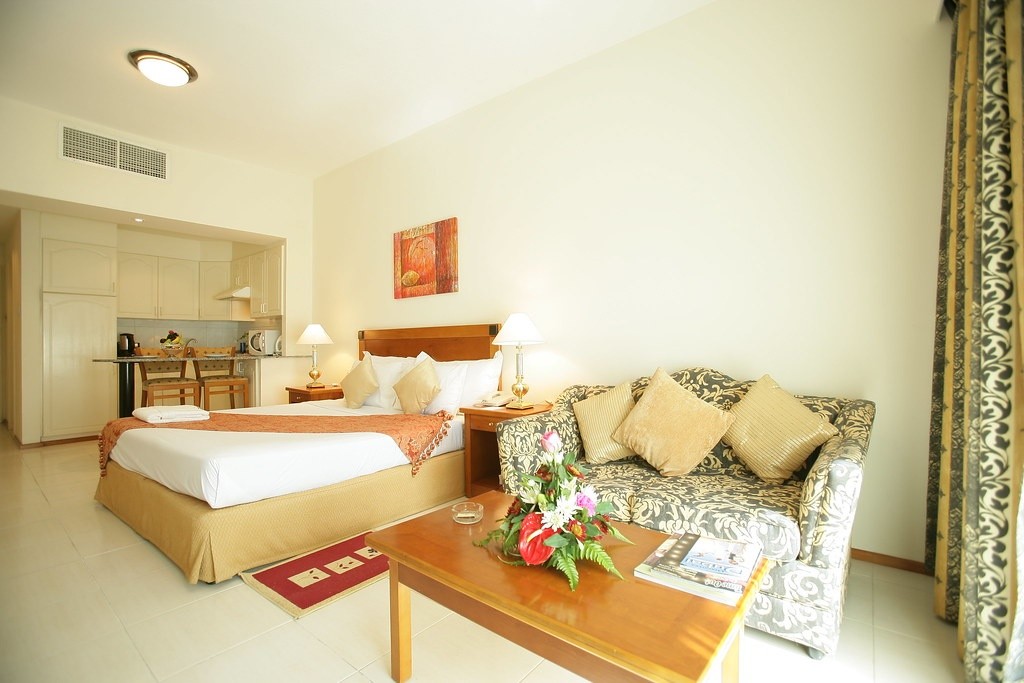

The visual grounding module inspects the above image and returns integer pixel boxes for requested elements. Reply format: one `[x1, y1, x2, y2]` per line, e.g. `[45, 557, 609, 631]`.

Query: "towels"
[131, 405, 211, 424]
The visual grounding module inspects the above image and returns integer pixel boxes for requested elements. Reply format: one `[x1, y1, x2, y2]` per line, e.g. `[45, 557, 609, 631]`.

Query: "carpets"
[237, 528, 394, 618]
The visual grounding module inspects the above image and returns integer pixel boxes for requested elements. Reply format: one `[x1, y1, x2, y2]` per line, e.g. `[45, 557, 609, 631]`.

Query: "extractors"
[217, 285, 250, 301]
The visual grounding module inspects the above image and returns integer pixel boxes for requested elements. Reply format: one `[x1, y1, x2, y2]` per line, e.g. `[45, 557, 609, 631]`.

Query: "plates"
[203, 354, 228, 357]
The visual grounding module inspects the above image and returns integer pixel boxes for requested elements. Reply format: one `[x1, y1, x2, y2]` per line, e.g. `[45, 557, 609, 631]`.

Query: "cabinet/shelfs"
[200, 261, 233, 318]
[118, 256, 197, 318]
[232, 259, 249, 287]
[248, 248, 285, 318]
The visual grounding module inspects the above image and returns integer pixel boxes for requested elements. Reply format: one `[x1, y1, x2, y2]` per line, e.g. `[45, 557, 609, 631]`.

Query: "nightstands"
[285, 384, 342, 404]
[460, 402, 542, 498]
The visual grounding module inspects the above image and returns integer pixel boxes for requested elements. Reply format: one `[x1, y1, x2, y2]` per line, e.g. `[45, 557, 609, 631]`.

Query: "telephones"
[481, 392, 515, 407]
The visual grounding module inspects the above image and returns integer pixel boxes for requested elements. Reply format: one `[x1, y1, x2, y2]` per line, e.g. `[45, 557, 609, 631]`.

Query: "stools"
[134, 345, 200, 407]
[190, 346, 248, 410]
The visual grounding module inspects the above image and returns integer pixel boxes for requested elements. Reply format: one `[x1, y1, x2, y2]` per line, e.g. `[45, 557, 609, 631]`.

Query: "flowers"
[473, 432, 637, 595]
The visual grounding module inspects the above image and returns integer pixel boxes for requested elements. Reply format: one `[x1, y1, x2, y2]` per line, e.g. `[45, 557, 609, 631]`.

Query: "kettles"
[119, 333, 136, 357]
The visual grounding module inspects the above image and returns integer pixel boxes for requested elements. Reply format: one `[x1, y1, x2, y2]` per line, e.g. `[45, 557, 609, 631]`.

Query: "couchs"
[500, 367, 875, 660]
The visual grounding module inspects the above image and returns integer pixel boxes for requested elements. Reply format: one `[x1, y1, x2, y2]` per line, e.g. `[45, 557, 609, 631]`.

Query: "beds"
[92, 322, 504, 587]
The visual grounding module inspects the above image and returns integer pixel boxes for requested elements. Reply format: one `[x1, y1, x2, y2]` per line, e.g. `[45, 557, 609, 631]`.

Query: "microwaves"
[248, 329, 280, 356]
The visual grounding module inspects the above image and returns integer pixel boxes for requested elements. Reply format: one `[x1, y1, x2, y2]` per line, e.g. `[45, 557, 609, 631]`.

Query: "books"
[632, 532, 765, 608]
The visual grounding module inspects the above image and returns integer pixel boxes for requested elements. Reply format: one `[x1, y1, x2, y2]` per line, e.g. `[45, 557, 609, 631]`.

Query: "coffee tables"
[366, 491, 773, 683]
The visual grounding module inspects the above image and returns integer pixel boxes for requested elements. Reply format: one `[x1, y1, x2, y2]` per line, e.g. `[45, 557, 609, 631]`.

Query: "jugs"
[275, 335, 282, 355]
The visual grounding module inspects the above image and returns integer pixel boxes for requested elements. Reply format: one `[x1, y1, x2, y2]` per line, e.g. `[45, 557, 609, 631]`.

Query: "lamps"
[493, 311, 555, 409]
[296, 324, 334, 388]
[127, 48, 199, 89]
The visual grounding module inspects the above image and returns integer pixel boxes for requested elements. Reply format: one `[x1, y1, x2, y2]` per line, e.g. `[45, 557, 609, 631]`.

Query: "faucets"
[183, 338, 199, 358]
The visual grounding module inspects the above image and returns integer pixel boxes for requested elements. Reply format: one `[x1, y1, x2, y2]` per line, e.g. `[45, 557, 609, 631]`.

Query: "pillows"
[353, 352, 418, 408]
[391, 351, 506, 413]
[720, 374, 840, 484]
[572, 379, 645, 464]
[612, 370, 734, 480]
[341, 357, 377, 408]
[393, 358, 438, 415]
